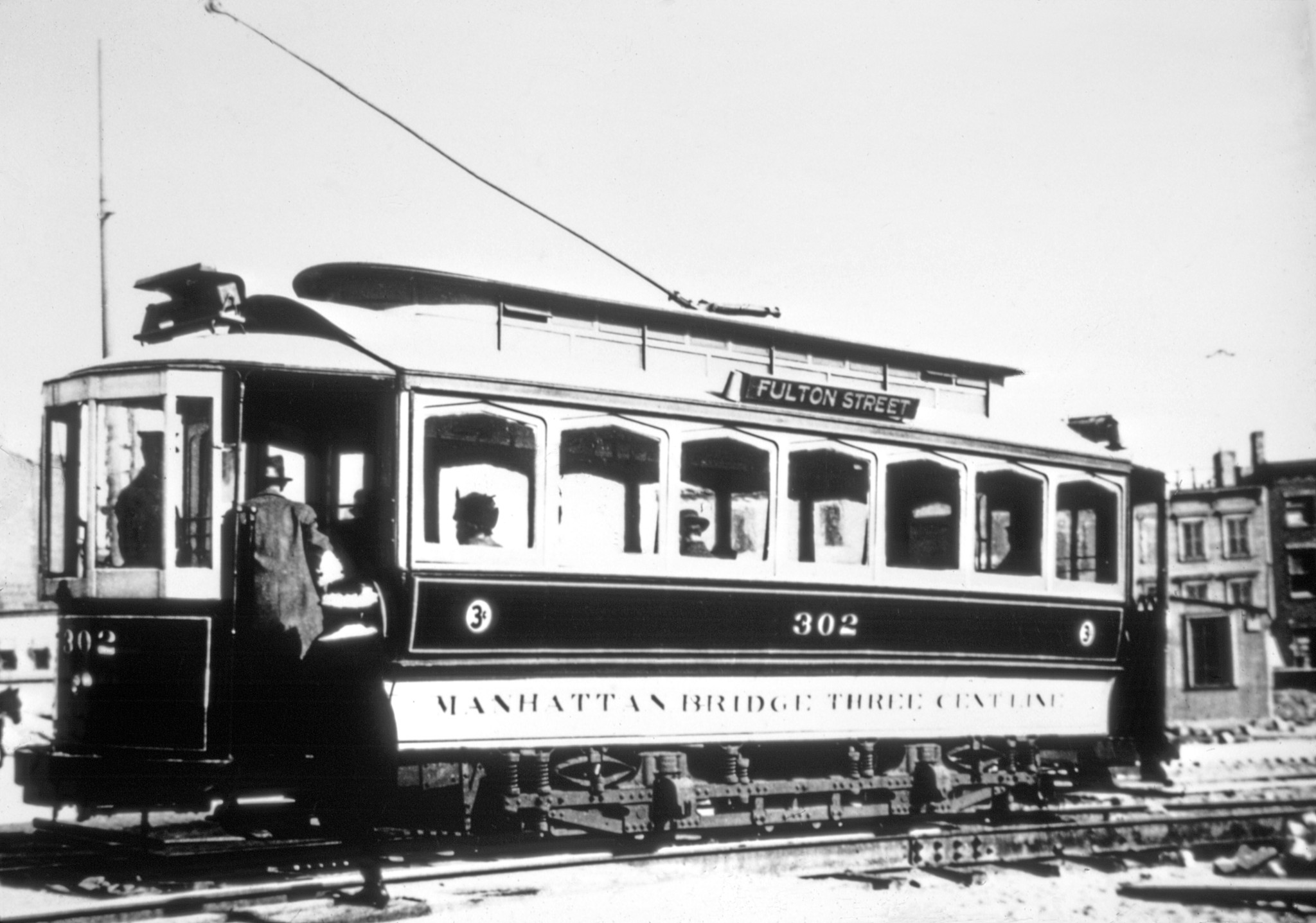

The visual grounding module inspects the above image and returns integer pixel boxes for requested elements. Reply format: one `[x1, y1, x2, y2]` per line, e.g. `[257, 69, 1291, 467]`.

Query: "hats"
[320, 578, 378, 611]
[254, 456, 292, 482]
[137, 430, 162, 448]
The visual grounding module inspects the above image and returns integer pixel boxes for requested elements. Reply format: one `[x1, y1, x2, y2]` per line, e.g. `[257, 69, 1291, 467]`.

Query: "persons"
[453, 492, 500, 546]
[292, 578, 398, 911]
[234, 457, 330, 659]
[115, 432, 190, 567]
[680, 509, 712, 557]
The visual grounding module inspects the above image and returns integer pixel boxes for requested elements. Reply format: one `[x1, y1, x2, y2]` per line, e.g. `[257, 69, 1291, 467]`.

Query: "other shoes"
[336, 883, 389, 906]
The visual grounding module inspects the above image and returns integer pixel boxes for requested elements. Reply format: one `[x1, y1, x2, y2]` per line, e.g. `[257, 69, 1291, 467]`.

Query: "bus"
[8, 2, 1170, 859]
